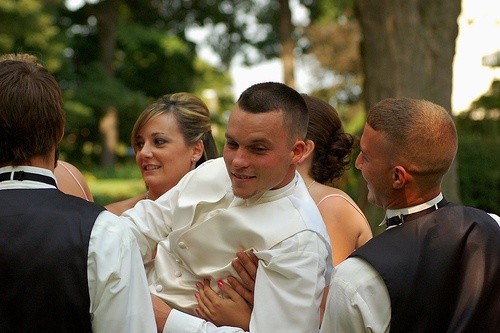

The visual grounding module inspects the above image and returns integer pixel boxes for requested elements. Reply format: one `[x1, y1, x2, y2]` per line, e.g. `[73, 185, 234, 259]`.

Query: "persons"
[193, 97, 500, 333]
[227, 137, 335, 330]
[117, 81, 327, 333]
[194, 92, 376, 333]
[0, 54, 97, 205]
[99, 91, 221, 281]
[0, 57, 158, 333]
